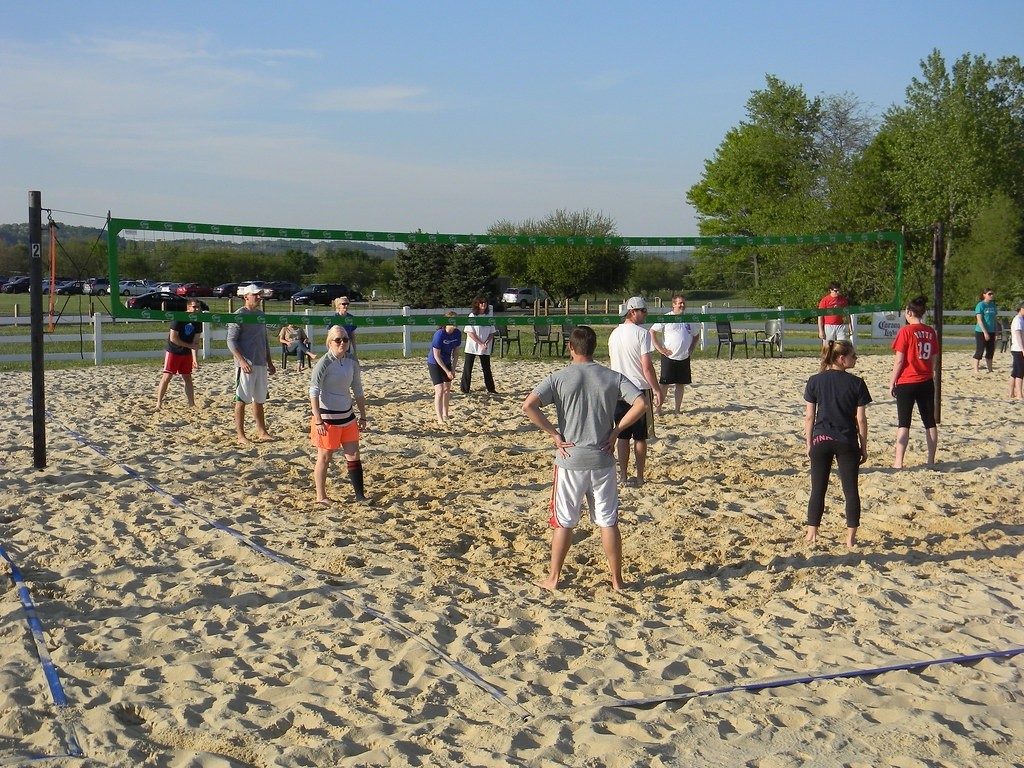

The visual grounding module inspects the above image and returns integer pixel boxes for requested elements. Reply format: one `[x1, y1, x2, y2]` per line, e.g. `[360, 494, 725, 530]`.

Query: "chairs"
[1000, 325, 1011, 352]
[754, 320, 783, 358]
[716, 322, 748, 361]
[491, 324, 522, 358]
[532, 324, 559, 357]
[282, 338, 311, 370]
[561, 324, 578, 356]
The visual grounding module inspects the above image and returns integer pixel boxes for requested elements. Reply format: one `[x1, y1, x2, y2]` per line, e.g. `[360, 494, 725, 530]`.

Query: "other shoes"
[355, 496, 373, 506]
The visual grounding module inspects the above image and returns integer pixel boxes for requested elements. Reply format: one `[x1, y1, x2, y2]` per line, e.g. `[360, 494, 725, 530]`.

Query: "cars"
[0, 276, 31, 294]
[177, 282, 213, 297]
[348, 288, 363, 301]
[212, 283, 238, 299]
[107, 281, 161, 295]
[126, 292, 210, 312]
[29, 280, 65, 294]
[55, 281, 84, 296]
[132, 280, 182, 296]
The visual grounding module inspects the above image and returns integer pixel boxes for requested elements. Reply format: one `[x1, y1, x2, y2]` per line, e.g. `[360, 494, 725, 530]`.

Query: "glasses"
[834, 290, 839, 292]
[333, 337, 351, 343]
[341, 303, 350, 305]
[988, 293, 994, 295]
[634, 308, 647, 313]
[192, 306, 200, 308]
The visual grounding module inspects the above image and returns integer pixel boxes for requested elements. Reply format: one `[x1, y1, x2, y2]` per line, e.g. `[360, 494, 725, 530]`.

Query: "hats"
[244, 284, 264, 295]
[618, 296, 648, 316]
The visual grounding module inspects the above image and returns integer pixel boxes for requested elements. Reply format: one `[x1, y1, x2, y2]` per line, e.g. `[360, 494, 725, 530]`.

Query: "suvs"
[82, 278, 109, 296]
[237, 281, 269, 299]
[502, 287, 549, 309]
[262, 282, 295, 300]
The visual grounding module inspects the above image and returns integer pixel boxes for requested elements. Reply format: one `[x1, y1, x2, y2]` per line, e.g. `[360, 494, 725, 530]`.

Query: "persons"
[608, 296, 661, 486]
[461, 295, 497, 393]
[523, 326, 647, 589]
[278, 323, 317, 373]
[804, 340, 873, 547]
[227, 284, 276, 444]
[889, 295, 937, 468]
[427, 312, 461, 424]
[1011, 302, 1024, 400]
[817, 282, 853, 345]
[157, 299, 203, 409]
[326, 296, 358, 355]
[308, 325, 374, 507]
[650, 295, 699, 414]
[973, 288, 997, 373]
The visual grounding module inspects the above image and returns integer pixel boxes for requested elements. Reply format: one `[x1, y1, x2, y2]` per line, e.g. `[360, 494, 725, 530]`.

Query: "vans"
[291, 284, 350, 306]
